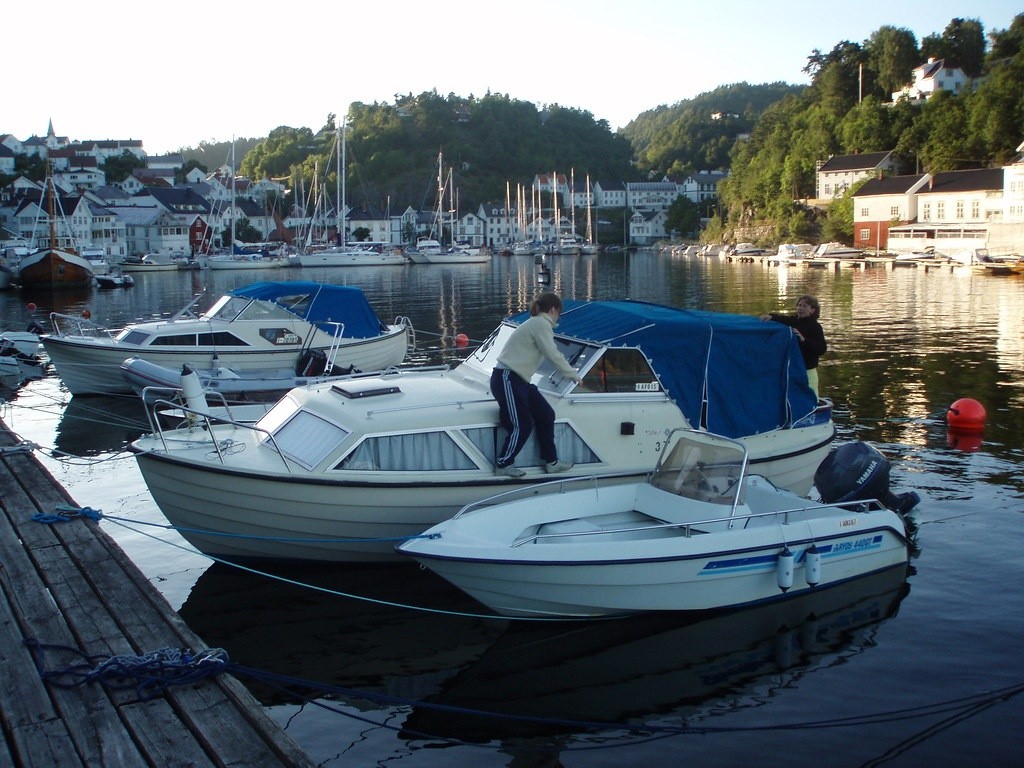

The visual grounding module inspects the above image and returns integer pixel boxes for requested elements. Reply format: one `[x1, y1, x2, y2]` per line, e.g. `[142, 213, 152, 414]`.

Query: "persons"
[483, 294, 582, 477]
[760, 296, 828, 406]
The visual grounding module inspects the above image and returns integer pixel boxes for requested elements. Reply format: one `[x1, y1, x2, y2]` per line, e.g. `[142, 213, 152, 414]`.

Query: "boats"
[93, 264, 135, 288]
[0, 279, 451, 432]
[650, 240, 1024, 274]
[127, 296, 839, 569]
[395, 429, 921, 623]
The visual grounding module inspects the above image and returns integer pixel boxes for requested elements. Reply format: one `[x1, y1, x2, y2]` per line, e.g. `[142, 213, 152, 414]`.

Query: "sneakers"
[495, 465, 526, 476]
[545, 460, 573, 473]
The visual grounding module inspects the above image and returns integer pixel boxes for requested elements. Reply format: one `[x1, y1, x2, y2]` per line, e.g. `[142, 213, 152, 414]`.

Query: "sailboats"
[13, 149, 94, 289]
[0, 115, 602, 271]
